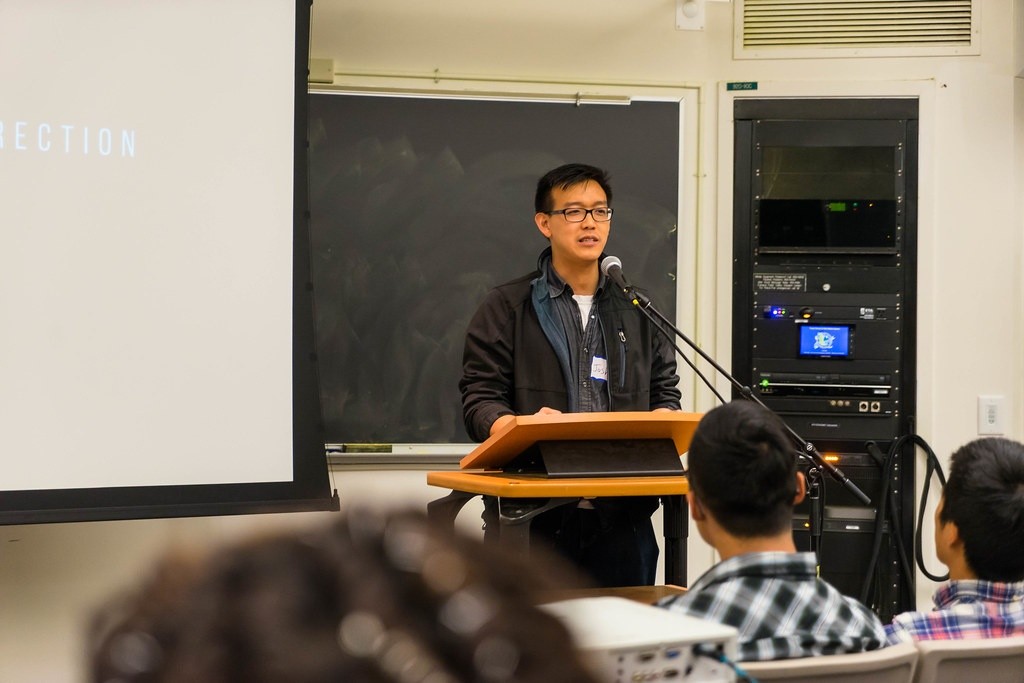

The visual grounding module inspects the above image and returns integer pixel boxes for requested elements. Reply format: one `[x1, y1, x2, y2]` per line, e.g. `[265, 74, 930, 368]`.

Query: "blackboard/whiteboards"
[301, 63, 704, 477]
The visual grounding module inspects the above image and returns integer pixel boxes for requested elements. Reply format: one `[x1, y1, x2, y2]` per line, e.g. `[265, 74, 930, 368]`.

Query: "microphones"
[601, 255, 640, 305]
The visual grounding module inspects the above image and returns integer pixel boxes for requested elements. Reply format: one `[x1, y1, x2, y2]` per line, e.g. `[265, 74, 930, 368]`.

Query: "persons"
[651, 400, 892, 661]
[84, 514, 594, 683]
[885, 438, 1024, 646]
[459, 164, 682, 588]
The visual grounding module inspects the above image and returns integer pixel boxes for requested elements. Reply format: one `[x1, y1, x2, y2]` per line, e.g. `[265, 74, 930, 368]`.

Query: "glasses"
[550, 208, 616, 223]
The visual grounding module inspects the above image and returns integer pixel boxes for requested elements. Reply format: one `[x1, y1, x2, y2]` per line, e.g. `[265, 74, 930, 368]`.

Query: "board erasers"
[342, 443, 393, 453]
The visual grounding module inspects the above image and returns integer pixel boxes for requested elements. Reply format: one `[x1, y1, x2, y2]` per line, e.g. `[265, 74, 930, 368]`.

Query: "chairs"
[732, 643, 919, 683]
[919, 636, 1024, 683]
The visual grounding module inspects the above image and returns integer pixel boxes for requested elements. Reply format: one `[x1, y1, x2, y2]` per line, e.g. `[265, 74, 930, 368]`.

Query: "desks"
[549, 583, 690, 606]
[533, 595, 739, 682]
[427, 469, 689, 589]
